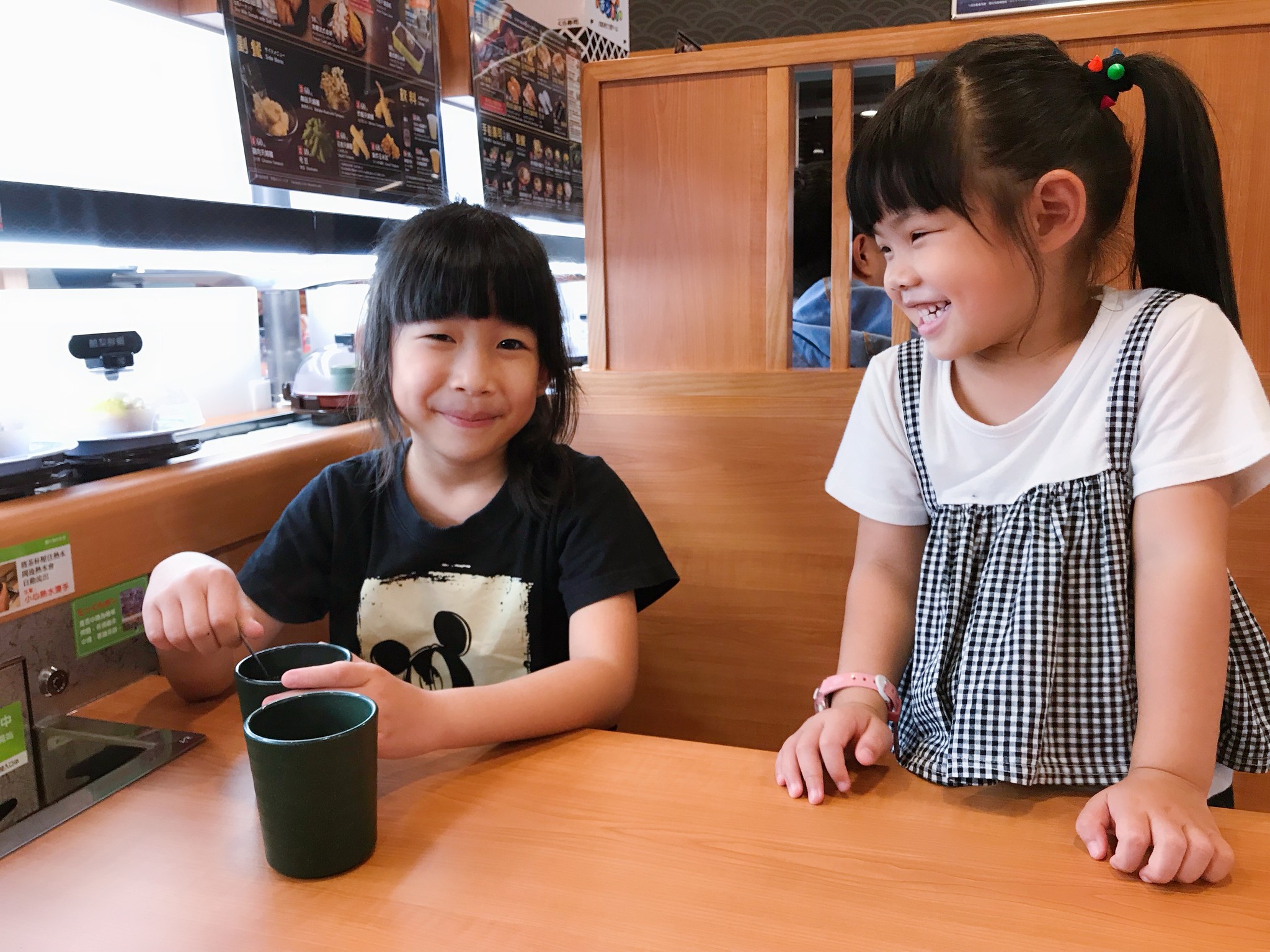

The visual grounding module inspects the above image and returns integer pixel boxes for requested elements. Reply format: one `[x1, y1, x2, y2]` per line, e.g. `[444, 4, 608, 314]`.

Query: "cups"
[430, 148, 440, 173]
[243, 689, 378, 879]
[234, 643, 353, 724]
[428, 116, 438, 140]
[329, 365, 356, 393]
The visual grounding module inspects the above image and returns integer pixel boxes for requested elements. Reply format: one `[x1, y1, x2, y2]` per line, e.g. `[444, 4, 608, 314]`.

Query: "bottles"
[402, 104, 411, 147]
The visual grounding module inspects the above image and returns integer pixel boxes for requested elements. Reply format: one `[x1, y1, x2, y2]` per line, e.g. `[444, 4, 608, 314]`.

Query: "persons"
[788, 164, 925, 369]
[141, 198, 683, 761]
[776, 30, 1270, 886]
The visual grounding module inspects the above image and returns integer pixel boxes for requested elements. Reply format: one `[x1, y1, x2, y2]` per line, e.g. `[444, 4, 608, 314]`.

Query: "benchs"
[546, 366, 1270, 817]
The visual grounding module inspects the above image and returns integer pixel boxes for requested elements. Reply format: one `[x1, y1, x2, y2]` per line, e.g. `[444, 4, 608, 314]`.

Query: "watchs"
[811, 671, 902, 727]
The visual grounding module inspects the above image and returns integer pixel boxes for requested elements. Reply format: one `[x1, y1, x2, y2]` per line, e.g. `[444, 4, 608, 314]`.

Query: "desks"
[0, 674, 1270, 952]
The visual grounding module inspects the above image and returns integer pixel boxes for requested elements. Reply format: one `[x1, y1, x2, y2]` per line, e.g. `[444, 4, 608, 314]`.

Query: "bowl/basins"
[515, 161, 531, 186]
[245, 91, 299, 156]
[320, 2, 367, 57]
[317, 71, 354, 116]
